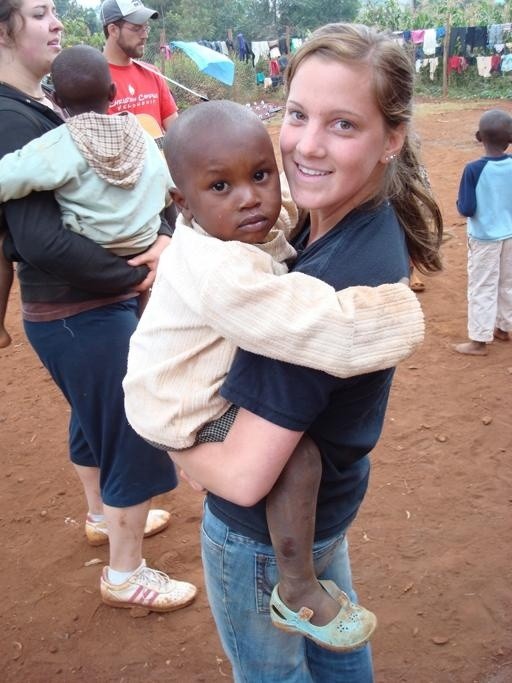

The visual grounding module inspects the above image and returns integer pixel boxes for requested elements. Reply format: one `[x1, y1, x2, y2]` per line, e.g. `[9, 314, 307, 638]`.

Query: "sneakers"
[408, 267, 425, 290]
[270, 580, 378, 652]
[101, 558, 198, 613]
[85, 508, 172, 545]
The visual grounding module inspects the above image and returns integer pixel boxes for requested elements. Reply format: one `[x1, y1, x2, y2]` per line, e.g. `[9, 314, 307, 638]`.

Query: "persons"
[450, 110, 512, 355]
[0, 45, 177, 348]
[99, 0, 180, 150]
[121, 99, 425, 653]
[167, 21, 443, 682]
[0, 0, 196, 614]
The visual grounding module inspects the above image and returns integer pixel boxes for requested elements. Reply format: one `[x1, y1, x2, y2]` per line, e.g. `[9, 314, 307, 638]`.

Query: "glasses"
[124, 25, 152, 34]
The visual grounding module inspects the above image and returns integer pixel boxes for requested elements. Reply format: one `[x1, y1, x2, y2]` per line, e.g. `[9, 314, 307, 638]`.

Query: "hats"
[100, 0, 159, 27]
